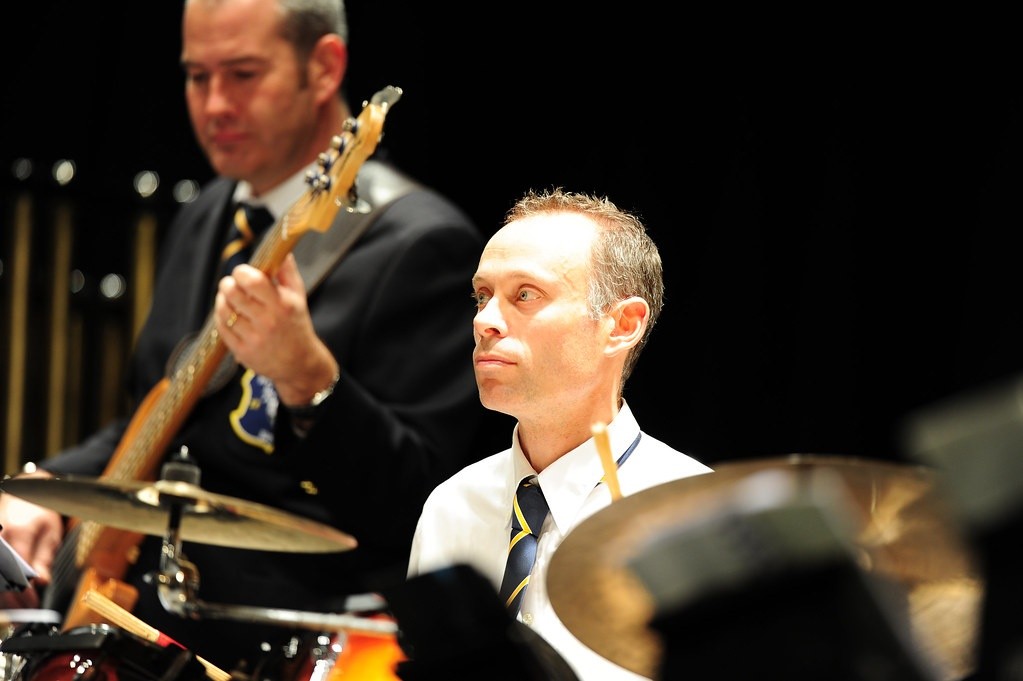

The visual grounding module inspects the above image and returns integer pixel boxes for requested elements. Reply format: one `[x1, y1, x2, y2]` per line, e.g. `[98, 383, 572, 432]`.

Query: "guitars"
[29, 82, 404, 651]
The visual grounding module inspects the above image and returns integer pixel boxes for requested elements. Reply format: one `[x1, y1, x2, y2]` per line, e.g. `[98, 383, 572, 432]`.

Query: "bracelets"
[310, 375, 339, 407]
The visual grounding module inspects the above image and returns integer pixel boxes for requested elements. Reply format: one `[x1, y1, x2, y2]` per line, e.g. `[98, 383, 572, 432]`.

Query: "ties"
[216, 202, 274, 284]
[498, 476, 550, 620]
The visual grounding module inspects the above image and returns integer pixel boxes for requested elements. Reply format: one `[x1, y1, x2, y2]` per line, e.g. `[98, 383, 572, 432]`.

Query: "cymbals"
[542, 454, 983, 680]
[172, 603, 401, 637]
[0, 475, 358, 554]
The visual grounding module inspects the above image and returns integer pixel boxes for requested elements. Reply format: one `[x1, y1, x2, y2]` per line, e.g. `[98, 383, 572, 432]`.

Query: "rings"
[226, 313, 239, 327]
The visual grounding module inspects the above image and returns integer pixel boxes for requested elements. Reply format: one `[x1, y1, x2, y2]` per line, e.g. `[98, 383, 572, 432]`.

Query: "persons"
[404, 191, 718, 681]
[0, 0, 518, 681]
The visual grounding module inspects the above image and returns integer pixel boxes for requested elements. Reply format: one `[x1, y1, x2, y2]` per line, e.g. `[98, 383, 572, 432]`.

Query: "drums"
[13, 623, 206, 681]
[300, 594, 412, 680]
